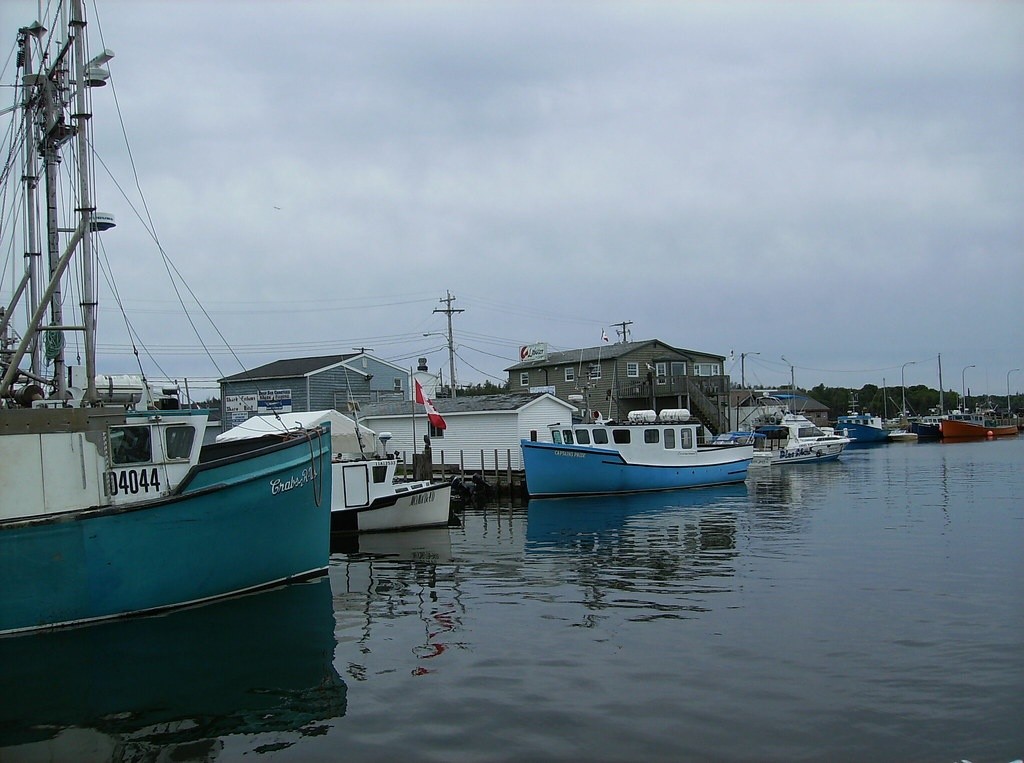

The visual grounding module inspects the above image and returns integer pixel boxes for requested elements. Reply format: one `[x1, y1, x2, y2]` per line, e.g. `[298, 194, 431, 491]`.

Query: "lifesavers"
[769, 416, 776, 425]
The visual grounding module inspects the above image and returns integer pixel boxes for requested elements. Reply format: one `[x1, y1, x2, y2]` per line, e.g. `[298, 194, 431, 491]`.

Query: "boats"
[0, 577, 349, 763]
[745, 391, 856, 466]
[327, 527, 455, 639]
[519, 332, 756, 500]
[0, 1, 338, 645]
[888, 430, 918, 441]
[909, 415, 949, 439]
[941, 413, 1018, 438]
[331, 357, 453, 536]
[519, 482, 747, 562]
[710, 431, 773, 468]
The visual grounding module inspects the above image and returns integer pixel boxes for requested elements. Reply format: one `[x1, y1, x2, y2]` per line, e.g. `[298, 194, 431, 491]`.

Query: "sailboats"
[832, 377, 892, 444]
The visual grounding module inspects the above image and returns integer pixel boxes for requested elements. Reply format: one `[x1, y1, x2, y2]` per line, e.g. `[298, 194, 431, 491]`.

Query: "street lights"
[901, 361, 915, 416]
[423, 332, 457, 399]
[962, 365, 975, 413]
[1007, 368, 1020, 412]
[741, 351, 761, 389]
[782, 358, 796, 414]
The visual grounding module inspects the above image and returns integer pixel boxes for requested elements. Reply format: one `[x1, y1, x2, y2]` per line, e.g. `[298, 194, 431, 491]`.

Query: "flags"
[602, 330, 609, 343]
[413, 377, 448, 431]
